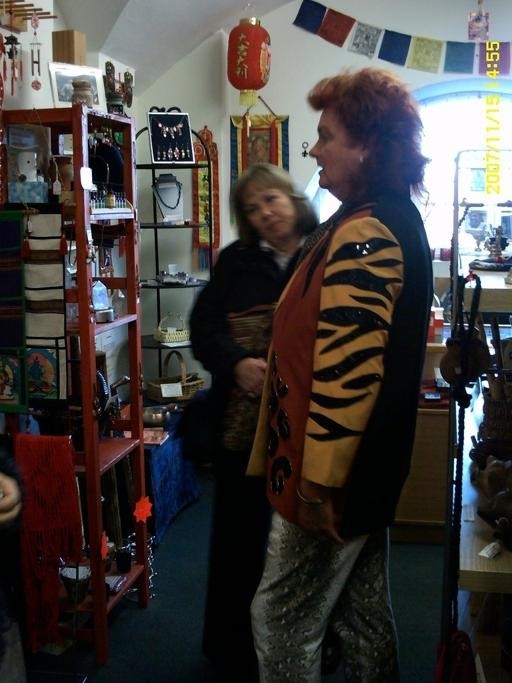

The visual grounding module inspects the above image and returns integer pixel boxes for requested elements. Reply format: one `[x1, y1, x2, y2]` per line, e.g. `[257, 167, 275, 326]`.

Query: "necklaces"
[153, 181, 182, 209]
[149, 114, 187, 139]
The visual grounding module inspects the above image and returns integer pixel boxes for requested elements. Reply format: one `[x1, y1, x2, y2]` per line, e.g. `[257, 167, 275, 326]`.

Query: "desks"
[121, 390, 202, 548]
[394, 323, 512, 524]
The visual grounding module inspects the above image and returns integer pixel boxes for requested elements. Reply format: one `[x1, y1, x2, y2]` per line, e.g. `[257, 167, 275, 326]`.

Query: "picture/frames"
[49, 61, 108, 113]
[147, 112, 196, 164]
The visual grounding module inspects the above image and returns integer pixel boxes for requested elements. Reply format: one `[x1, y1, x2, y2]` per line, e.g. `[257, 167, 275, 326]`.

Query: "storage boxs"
[52, 29, 87, 64]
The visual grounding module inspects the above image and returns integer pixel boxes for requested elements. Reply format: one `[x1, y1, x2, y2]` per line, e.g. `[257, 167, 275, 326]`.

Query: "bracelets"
[295, 488, 326, 507]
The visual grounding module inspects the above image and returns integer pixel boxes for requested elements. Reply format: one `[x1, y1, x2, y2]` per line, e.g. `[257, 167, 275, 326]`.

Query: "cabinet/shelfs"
[458, 269, 512, 594]
[133, 106, 213, 376]
[0, 104, 149, 664]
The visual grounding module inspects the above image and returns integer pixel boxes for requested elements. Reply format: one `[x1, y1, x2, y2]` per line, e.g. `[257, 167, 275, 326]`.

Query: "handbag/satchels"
[173, 388, 259, 480]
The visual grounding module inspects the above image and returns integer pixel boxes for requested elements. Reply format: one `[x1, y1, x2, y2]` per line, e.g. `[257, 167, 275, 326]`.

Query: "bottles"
[107, 93, 123, 114]
[71, 80, 92, 108]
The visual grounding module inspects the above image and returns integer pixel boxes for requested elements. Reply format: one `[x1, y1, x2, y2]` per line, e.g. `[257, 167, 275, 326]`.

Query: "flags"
[293, 0, 510, 78]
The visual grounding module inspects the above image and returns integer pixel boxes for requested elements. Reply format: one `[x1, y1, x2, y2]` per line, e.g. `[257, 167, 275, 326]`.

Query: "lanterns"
[227, 17, 273, 106]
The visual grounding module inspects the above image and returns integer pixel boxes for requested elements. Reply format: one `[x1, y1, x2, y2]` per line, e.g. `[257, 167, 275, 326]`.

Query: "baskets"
[148, 349, 205, 401]
[154, 310, 190, 342]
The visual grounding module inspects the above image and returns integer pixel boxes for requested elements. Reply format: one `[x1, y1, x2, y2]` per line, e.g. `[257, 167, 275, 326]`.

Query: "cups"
[61, 566, 91, 600]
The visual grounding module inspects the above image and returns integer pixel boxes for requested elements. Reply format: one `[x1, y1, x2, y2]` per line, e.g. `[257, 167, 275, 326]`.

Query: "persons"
[182, 165, 322, 682]
[2, 456, 32, 682]
[244, 61, 435, 683]
